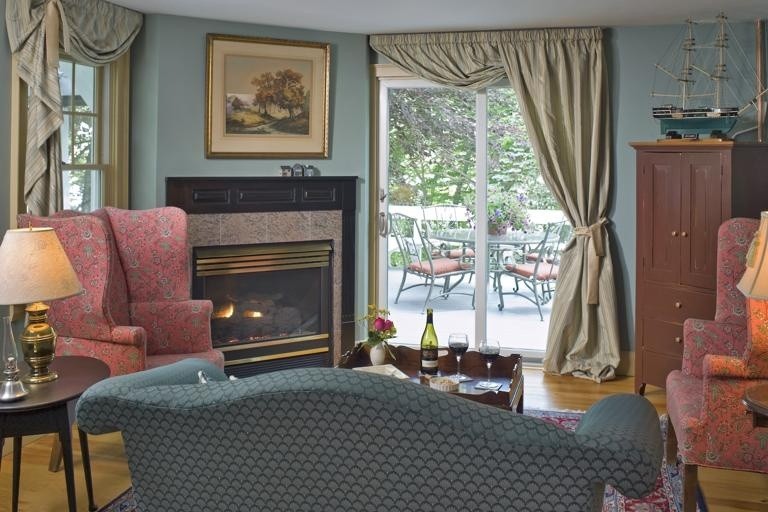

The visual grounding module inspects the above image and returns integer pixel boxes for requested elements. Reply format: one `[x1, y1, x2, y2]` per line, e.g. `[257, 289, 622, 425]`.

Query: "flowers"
[467, 185, 532, 234]
[355, 305, 396, 360]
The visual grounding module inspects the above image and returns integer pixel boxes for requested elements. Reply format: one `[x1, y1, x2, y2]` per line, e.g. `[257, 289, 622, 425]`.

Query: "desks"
[429, 234, 556, 299]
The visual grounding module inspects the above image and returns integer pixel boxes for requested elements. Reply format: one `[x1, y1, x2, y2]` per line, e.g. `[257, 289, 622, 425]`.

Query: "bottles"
[420, 308, 439, 378]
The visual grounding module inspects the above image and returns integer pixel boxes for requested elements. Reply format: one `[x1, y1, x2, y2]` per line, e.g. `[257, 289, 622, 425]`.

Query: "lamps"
[0, 223, 85, 384]
[735, 212, 768, 300]
[0, 316, 27, 402]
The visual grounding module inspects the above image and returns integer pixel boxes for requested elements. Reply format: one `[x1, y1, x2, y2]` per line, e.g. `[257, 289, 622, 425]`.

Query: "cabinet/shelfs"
[628, 138, 768, 396]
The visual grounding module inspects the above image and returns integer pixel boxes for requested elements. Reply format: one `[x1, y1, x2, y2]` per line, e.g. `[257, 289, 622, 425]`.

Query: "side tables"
[0, 355, 109, 511]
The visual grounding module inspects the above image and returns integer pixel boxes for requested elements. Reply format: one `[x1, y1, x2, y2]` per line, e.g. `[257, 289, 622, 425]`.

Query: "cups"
[479, 339, 499, 388]
[449, 332, 468, 382]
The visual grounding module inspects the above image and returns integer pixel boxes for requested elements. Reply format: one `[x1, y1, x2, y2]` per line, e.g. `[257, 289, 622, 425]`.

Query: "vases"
[370, 343, 386, 366]
[487, 224, 507, 237]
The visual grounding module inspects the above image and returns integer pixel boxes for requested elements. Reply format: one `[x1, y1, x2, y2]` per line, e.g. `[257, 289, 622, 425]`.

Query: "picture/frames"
[203, 33, 332, 159]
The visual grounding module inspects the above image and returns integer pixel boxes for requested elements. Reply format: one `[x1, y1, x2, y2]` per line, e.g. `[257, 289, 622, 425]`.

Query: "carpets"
[100, 408, 680, 512]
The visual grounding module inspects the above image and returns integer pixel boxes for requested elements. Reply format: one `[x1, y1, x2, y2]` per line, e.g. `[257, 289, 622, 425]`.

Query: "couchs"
[17, 206, 223, 475]
[76, 360, 664, 512]
[666, 217, 768, 512]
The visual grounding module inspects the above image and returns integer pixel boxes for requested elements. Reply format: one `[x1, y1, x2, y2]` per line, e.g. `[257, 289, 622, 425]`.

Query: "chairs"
[494, 222, 564, 322]
[389, 213, 477, 316]
[513, 221, 556, 304]
[432, 206, 478, 301]
